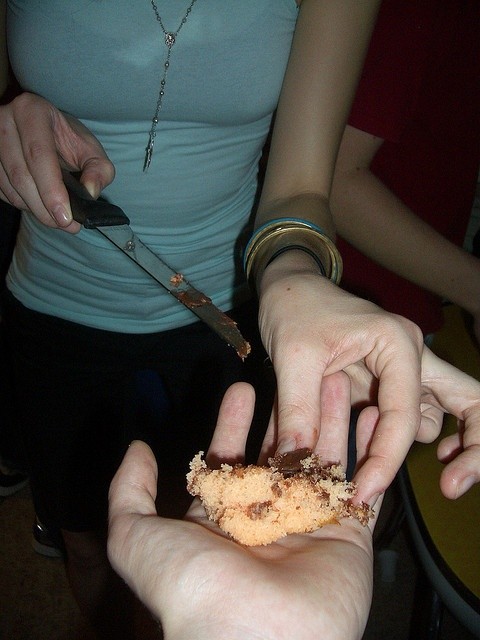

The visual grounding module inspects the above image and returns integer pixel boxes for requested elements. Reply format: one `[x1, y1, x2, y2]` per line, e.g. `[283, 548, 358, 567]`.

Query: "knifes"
[59, 164, 253, 360]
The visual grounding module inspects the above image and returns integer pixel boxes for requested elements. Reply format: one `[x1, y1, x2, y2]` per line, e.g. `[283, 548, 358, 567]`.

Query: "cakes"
[185, 450, 375, 548]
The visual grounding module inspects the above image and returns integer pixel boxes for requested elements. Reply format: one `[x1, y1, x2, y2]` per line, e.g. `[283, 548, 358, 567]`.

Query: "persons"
[1, 0, 478, 640]
[327, 0, 480, 345]
[101, 368, 388, 640]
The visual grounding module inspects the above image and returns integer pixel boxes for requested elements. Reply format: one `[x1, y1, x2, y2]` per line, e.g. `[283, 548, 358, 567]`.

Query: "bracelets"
[239, 216, 344, 290]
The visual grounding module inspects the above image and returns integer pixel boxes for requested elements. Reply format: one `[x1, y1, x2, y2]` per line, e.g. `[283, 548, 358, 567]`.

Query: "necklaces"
[142, 1, 196, 174]
